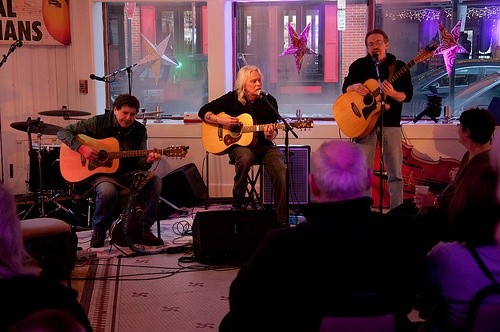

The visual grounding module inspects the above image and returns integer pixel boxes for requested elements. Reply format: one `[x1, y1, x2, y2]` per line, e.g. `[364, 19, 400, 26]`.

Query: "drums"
[28, 144, 67, 195]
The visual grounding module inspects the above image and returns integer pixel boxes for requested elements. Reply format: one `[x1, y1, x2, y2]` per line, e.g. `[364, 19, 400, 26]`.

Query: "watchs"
[434, 198, 440, 208]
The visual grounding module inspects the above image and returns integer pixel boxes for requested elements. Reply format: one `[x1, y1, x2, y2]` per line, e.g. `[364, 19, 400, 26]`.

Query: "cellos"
[372, 140, 462, 209]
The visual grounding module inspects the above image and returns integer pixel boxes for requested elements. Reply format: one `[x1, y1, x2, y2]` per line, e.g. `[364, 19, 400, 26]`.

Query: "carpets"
[64, 257, 122, 332]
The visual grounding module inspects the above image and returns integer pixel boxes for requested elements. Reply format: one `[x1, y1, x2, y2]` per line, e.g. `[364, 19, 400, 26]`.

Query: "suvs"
[110, 53, 248, 113]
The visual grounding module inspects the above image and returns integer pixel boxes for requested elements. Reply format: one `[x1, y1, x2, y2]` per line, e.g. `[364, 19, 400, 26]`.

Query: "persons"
[386, 108, 495, 232]
[427, 165, 500, 332]
[342, 29, 414, 208]
[196, 66, 289, 227]
[218, 141, 452, 332]
[0, 185, 93, 332]
[56, 94, 164, 248]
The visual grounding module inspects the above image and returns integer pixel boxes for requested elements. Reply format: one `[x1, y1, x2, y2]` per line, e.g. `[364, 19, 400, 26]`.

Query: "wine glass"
[442, 106, 453, 123]
[154, 105, 163, 123]
[296, 107, 302, 121]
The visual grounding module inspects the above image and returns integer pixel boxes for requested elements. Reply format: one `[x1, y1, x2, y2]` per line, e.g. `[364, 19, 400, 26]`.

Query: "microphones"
[15, 40, 23, 47]
[257, 90, 269, 97]
[372, 53, 380, 65]
[89, 73, 107, 83]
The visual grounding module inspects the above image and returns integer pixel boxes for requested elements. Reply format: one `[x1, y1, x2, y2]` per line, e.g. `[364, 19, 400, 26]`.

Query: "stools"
[229, 155, 264, 211]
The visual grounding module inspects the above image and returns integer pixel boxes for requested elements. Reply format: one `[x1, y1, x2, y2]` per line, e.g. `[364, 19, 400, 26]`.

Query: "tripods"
[16, 143, 72, 220]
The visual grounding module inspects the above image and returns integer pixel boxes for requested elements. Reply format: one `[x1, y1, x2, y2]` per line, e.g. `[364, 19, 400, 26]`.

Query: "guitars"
[333, 44, 438, 139]
[60, 133, 189, 183]
[201, 112, 314, 156]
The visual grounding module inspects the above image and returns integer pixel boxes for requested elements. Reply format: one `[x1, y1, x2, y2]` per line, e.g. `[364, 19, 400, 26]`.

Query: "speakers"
[30, 148, 76, 196]
[262, 144, 310, 207]
[160, 162, 207, 219]
[187, 209, 292, 266]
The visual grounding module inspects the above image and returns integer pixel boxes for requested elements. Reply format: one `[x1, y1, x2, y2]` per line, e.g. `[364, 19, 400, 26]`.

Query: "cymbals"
[38, 109, 91, 116]
[137, 111, 163, 115]
[10, 121, 63, 135]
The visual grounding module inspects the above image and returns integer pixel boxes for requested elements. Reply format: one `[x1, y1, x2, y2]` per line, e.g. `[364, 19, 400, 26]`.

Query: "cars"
[437, 70, 500, 120]
[401, 60, 500, 117]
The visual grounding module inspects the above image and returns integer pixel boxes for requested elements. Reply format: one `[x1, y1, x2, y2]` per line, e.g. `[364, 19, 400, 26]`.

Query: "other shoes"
[90, 230, 106, 248]
[136, 228, 164, 245]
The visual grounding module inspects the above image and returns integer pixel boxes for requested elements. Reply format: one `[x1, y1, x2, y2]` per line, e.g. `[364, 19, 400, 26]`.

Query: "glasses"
[367, 43, 383, 48]
[457, 124, 461, 127]
